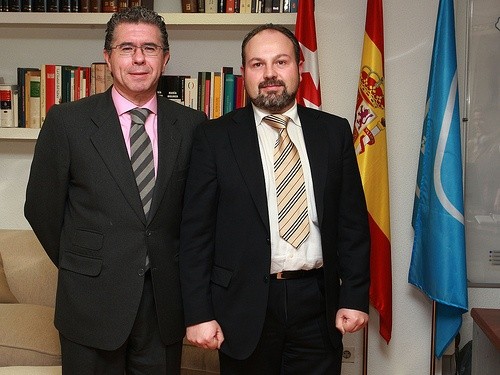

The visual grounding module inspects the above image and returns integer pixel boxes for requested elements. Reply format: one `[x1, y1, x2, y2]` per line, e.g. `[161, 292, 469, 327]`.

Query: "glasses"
[110, 45, 165, 56]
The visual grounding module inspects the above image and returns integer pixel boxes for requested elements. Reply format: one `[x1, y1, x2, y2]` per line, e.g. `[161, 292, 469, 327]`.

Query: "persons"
[181, 24, 372, 375]
[23, 6, 207, 375]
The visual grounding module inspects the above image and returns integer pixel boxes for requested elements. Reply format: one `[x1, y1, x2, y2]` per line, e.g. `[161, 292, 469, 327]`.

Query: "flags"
[408, 0, 469, 361]
[295, 0, 322, 111]
[352, 0, 393, 344]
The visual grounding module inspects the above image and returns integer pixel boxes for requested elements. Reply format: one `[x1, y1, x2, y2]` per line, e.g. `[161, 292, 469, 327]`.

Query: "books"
[0, 63, 248, 129]
[0, 0, 300, 13]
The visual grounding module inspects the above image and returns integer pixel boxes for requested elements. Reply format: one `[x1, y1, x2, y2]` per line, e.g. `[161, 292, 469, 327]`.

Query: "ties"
[128, 107, 156, 220]
[261, 113, 311, 250]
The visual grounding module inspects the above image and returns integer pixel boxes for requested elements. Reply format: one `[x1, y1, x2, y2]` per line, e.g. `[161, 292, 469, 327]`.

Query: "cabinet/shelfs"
[0, 0, 298, 140]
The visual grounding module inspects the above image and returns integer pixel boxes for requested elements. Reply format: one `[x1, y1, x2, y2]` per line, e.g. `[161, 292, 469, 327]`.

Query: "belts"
[271, 269, 322, 281]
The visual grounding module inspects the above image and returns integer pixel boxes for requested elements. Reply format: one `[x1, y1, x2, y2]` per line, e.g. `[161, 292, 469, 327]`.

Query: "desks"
[464, 308, 500, 375]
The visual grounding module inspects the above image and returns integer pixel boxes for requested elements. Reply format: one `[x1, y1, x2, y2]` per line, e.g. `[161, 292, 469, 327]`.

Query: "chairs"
[1, 230, 88, 367]
